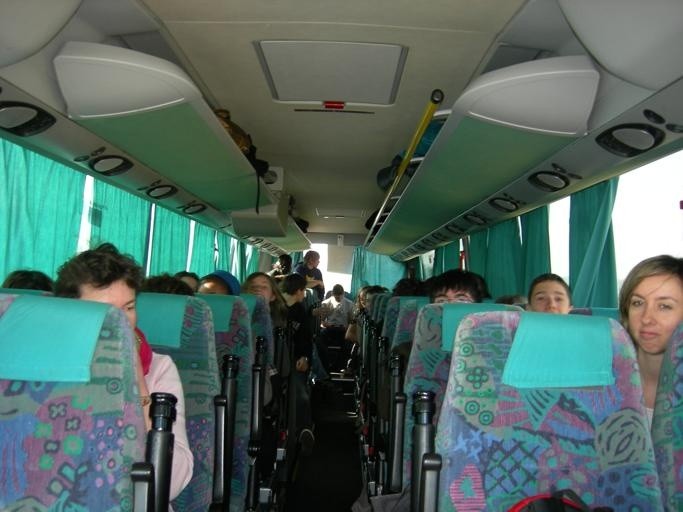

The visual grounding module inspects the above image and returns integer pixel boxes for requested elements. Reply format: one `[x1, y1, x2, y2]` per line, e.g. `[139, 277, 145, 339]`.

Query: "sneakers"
[294, 430, 313, 467]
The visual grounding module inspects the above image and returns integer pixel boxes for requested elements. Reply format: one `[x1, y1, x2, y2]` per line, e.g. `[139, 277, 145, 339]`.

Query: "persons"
[365, 269, 574, 328]
[48, 242, 195, 511]
[612, 254, 683, 438]
[3, 271, 53, 291]
[321, 284, 371, 375]
[241, 250, 324, 453]
[140, 269, 241, 302]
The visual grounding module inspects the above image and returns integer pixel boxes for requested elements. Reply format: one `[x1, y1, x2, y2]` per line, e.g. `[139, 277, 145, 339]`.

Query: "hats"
[209, 269, 242, 295]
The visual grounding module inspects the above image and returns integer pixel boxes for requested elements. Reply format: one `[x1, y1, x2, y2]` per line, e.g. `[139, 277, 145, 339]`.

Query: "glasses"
[434, 297, 471, 304]
